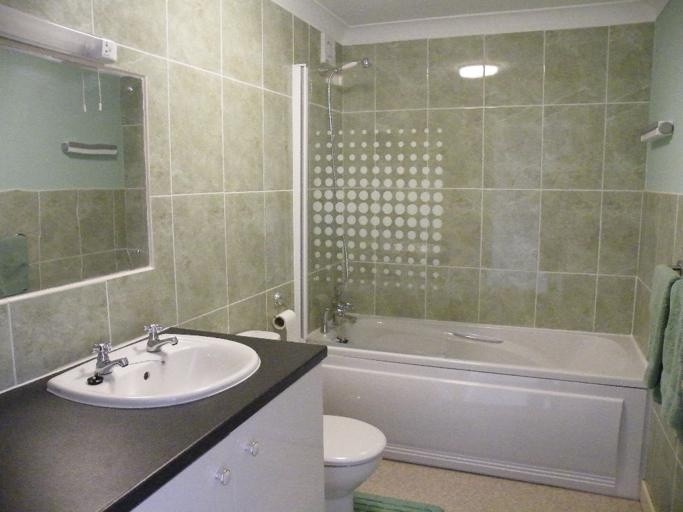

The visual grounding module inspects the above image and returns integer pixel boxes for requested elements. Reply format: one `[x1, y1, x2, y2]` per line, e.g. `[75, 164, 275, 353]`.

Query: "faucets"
[142, 323, 179, 354]
[319, 308, 345, 333]
[92, 342, 128, 375]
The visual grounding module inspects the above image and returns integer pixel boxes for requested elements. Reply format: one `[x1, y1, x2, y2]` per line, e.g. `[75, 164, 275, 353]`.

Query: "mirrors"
[0, 45, 155, 305]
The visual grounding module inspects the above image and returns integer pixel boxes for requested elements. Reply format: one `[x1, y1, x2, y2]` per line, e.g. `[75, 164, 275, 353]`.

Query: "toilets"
[235, 330, 387, 512]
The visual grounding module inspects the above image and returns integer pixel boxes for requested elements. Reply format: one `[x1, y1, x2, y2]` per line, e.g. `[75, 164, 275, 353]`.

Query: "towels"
[660, 280, 683, 431]
[644, 264, 679, 404]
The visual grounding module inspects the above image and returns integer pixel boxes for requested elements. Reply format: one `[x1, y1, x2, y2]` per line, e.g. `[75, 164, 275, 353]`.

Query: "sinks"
[46, 333, 262, 410]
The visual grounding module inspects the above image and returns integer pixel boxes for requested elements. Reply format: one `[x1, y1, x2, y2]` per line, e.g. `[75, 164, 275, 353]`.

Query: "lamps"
[0, 4, 117, 63]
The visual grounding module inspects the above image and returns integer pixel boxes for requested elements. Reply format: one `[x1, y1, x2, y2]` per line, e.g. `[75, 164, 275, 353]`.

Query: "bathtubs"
[305, 313, 649, 499]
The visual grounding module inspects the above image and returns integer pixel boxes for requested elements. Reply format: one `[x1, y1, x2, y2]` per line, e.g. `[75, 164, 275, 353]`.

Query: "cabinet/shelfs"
[130, 361, 325, 512]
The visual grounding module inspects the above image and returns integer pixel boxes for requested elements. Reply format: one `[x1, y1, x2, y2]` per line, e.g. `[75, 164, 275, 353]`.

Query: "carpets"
[355, 490, 444, 512]
